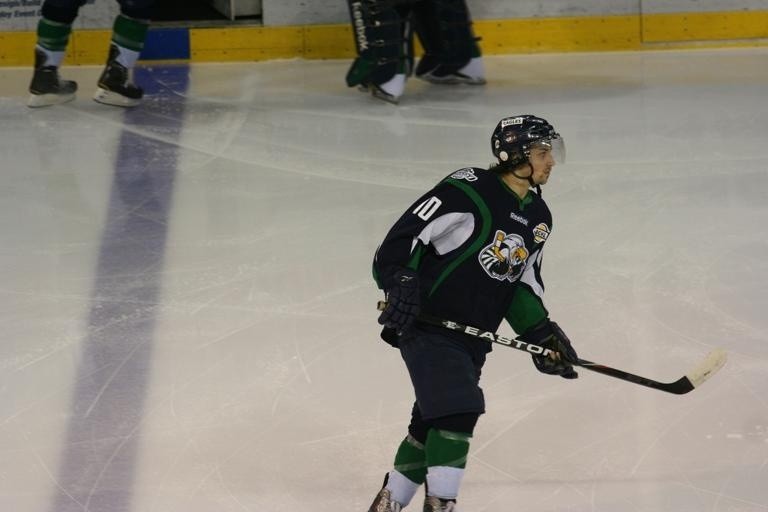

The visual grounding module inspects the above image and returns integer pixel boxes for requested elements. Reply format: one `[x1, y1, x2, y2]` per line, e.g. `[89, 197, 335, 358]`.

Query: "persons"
[345, 0, 489, 97]
[27, 0, 154, 99]
[368, 114, 578, 512]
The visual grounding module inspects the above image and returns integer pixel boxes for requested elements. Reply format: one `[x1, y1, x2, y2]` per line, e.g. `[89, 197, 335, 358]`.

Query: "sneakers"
[28, 65, 77, 96]
[419, 54, 486, 81]
[97, 60, 143, 99]
[355, 72, 407, 98]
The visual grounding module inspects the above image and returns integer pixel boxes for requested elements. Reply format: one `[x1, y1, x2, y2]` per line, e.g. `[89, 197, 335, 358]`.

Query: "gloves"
[378, 268, 423, 334]
[515, 321, 580, 379]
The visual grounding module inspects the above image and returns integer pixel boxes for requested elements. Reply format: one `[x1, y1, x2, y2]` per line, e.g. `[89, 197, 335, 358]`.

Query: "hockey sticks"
[378, 300, 727, 394]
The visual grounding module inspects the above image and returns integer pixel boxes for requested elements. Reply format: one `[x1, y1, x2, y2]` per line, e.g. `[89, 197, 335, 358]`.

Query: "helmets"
[491, 113, 565, 170]
